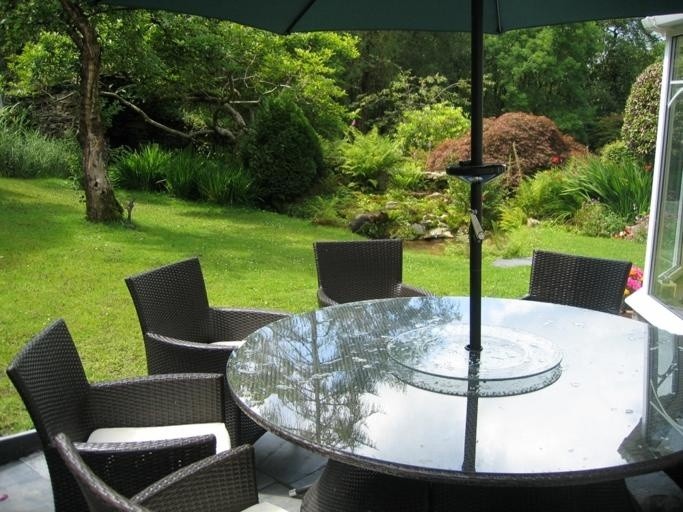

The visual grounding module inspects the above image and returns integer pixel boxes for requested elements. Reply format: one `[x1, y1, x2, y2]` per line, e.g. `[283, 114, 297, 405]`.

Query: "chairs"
[311, 238, 426, 307]
[519, 248, 633, 315]
[122, 256, 290, 450]
[5, 316, 261, 511]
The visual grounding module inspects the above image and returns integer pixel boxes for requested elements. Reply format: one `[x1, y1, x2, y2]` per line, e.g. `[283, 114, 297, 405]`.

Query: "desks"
[227, 294, 682, 511]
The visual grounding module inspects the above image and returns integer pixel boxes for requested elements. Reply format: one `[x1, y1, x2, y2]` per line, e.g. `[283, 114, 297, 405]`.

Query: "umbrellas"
[94, 0, 682, 351]
